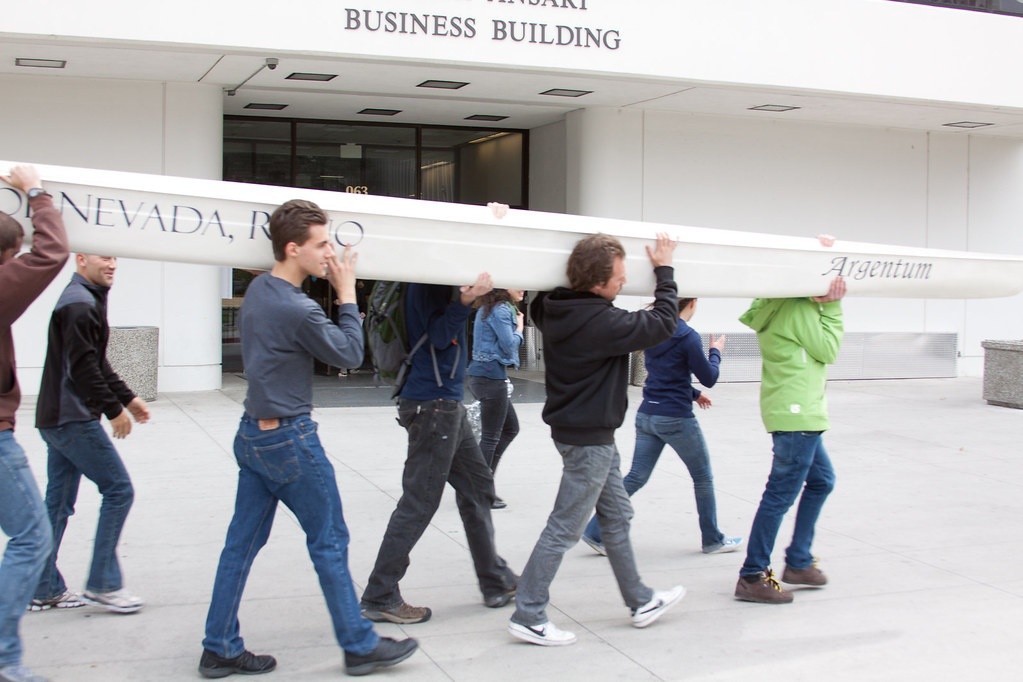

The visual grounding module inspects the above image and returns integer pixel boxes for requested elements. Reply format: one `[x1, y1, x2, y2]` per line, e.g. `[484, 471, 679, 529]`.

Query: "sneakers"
[26, 590, 86, 611]
[84, 591, 144, 613]
[345, 637, 419, 676]
[735, 569, 793, 604]
[508, 620, 578, 646]
[581, 536, 607, 556]
[361, 602, 431, 624]
[707, 537, 743, 554]
[489, 576, 520, 608]
[781, 565, 826, 585]
[631, 585, 688, 628]
[199, 648, 276, 676]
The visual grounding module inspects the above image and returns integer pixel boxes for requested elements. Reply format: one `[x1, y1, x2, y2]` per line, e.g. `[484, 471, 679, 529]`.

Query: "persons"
[466, 288, 523, 510]
[580, 297, 741, 556]
[356, 201, 520, 624]
[198, 199, 416, 681]
[23, 252, 149, 612]
[508, 230, 687, 646]
[733, 235, 847, 605]
[0, 165, 69, 682]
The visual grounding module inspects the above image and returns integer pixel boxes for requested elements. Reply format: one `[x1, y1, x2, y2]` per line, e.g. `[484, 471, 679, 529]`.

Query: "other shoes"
[491, 498, 506, 508]
[338, 372, 348, 376]
[0, 666, 50, 682]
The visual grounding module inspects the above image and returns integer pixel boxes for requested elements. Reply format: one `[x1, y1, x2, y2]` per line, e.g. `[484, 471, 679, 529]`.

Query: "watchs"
[26, 188, 45, 200]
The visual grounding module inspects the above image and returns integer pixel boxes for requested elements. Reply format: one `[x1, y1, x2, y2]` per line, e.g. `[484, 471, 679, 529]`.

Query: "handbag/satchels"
[349, 369, 360, 374]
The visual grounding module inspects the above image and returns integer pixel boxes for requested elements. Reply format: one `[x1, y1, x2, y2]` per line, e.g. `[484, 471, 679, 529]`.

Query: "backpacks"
[365, 281, 427, 387]
